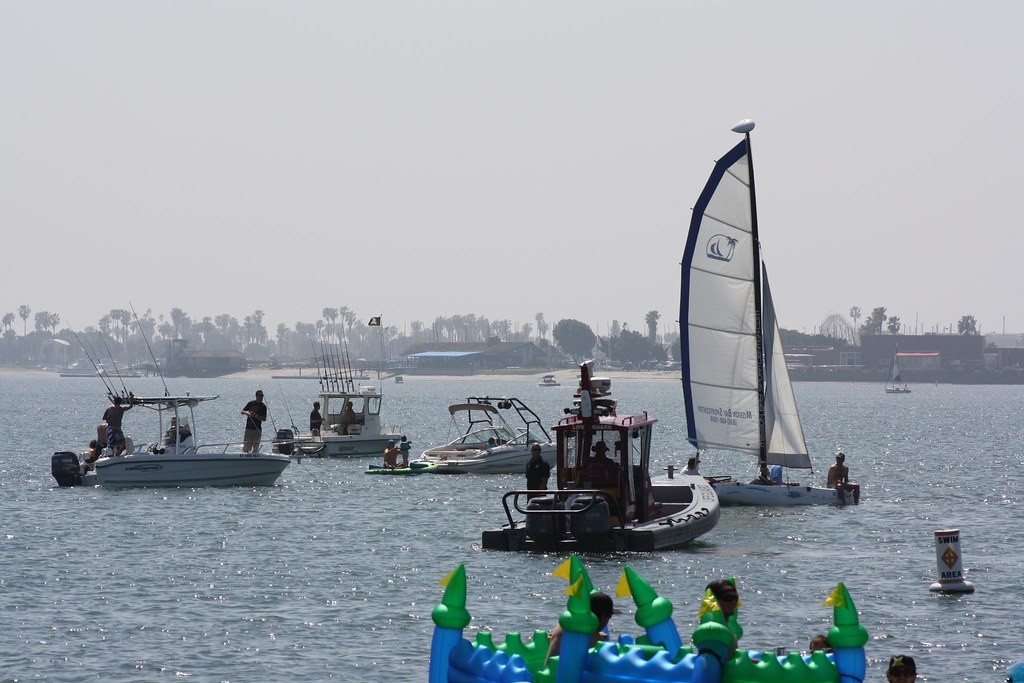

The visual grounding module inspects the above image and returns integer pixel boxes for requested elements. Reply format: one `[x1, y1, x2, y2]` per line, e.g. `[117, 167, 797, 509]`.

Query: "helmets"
[530, 443, 542, 451]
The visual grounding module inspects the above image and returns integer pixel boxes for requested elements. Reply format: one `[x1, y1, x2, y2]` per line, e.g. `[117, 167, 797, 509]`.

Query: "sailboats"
[676, 117, 856, 508]
[885, 342, 912, 394]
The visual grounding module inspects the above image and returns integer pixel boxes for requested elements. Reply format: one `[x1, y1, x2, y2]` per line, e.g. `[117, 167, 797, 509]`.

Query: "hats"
[835, 451, 846, 460]
[591, 441, 610, 452]
[889, 654, 917, 674]
[686, 457, 700, 465]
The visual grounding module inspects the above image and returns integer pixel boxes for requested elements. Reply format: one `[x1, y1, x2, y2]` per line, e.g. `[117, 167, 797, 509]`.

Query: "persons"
[706, 578, 760, 663]
[768, 464, 784, 486]
[827, 451, 860, 506]
[165, 417, 192, 447]
[886, 655, 917, 683]
[485, 437, 500, 450]
[809, 635, 834, 654]
[88, 440, 97, 458]
[543, 592, 613, 669]
[584, 441, 620, 489]
[103, 394, 134, 458]
[525, 443, 550, 505]
[342, 402, 356, 435]
[384, 436, 409, 469]
[680, 457, 701, 475]
[241, 390, 267, 453]
[310, 402, 326, 437]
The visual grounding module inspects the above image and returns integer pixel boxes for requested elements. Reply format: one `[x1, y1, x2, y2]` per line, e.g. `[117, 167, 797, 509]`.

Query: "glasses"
[893, 669, 914, 677]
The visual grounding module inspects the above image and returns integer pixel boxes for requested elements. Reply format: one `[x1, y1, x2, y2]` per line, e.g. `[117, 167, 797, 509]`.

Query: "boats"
[365, 394, 574, 476]
[51, 391, 291, 489]
[481, 355, 722, 554]
[271, 331, 403, 457]
[537, 375, 561, 387]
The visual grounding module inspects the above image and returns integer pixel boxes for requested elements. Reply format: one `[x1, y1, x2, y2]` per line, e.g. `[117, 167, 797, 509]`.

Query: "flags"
[368, 317, 380, 326]
